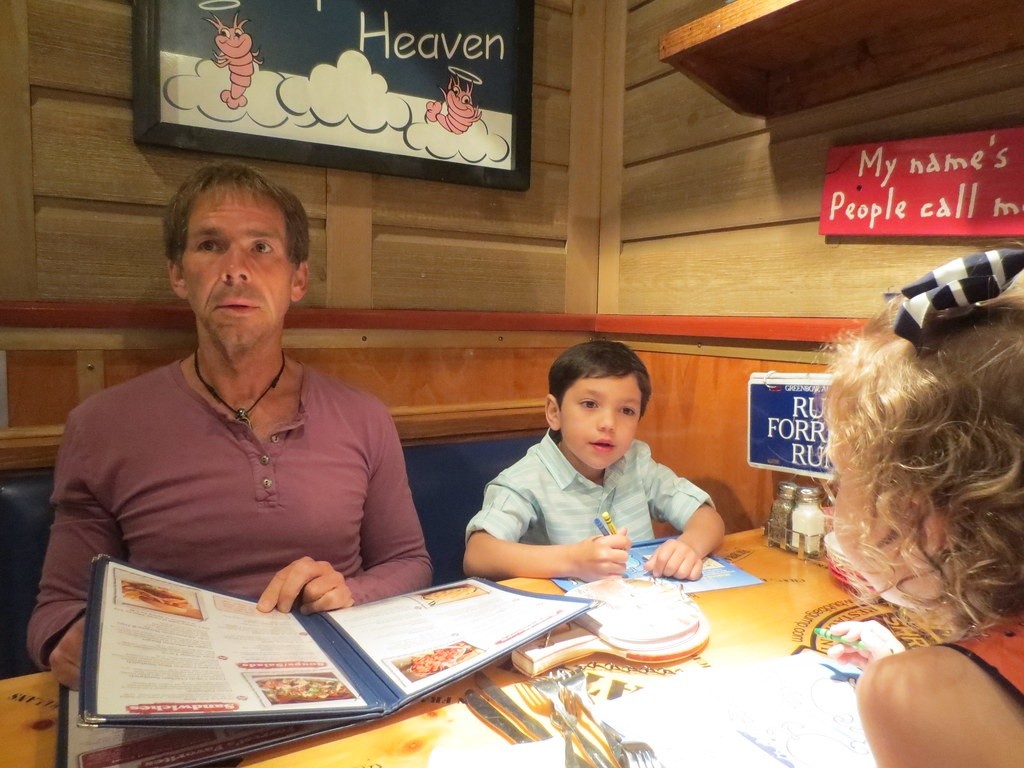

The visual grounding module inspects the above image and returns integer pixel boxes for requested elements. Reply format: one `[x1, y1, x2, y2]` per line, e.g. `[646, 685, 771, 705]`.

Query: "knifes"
[465, 687, 532, 744]
[474, 671, 552, 740]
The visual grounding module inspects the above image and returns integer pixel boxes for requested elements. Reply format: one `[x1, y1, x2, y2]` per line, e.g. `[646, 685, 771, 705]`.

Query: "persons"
[26, 165, 433, 691]
[827, 247, 1024, 767]
[457, 341, 724, 581]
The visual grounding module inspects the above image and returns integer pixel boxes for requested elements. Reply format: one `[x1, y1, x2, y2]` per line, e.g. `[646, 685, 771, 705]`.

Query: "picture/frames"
[131, 0, 535, 192]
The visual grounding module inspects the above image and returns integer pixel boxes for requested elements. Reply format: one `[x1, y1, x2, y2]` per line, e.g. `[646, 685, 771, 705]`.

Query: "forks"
[515, 664, 665, 767]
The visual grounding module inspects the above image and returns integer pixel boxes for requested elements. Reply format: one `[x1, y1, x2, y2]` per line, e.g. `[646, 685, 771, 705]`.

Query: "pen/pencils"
[595, 518, 609, 536]
[602, 511, 617, 535]
[813, 627, 865, 650]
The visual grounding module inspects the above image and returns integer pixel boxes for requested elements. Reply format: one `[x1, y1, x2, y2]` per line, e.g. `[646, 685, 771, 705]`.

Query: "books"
[56, 553, 606, 768]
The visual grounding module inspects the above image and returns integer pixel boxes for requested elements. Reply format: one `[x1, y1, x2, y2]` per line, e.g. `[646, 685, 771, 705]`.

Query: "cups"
[824, 531, 878, 600]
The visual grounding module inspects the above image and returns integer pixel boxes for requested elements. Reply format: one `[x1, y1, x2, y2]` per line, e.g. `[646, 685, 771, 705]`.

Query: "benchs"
[0, 436, 543, 679]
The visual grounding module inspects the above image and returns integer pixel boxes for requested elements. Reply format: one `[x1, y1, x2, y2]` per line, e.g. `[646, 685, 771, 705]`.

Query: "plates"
[409, 646, 465, 677]
[254, 677, 338, 692]
[421, 586, 476, 601]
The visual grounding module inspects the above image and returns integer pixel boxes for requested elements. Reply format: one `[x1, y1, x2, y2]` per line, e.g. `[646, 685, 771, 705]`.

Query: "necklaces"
[195, 347, 285, 430]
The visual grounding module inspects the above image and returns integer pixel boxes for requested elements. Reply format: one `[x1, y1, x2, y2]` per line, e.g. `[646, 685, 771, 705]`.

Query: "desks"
[0, 527, 960, 768]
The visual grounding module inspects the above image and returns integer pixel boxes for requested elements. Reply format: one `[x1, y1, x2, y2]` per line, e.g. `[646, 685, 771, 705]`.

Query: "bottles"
[768, 482, 799, 543]
[787, 486, 825, 556]
